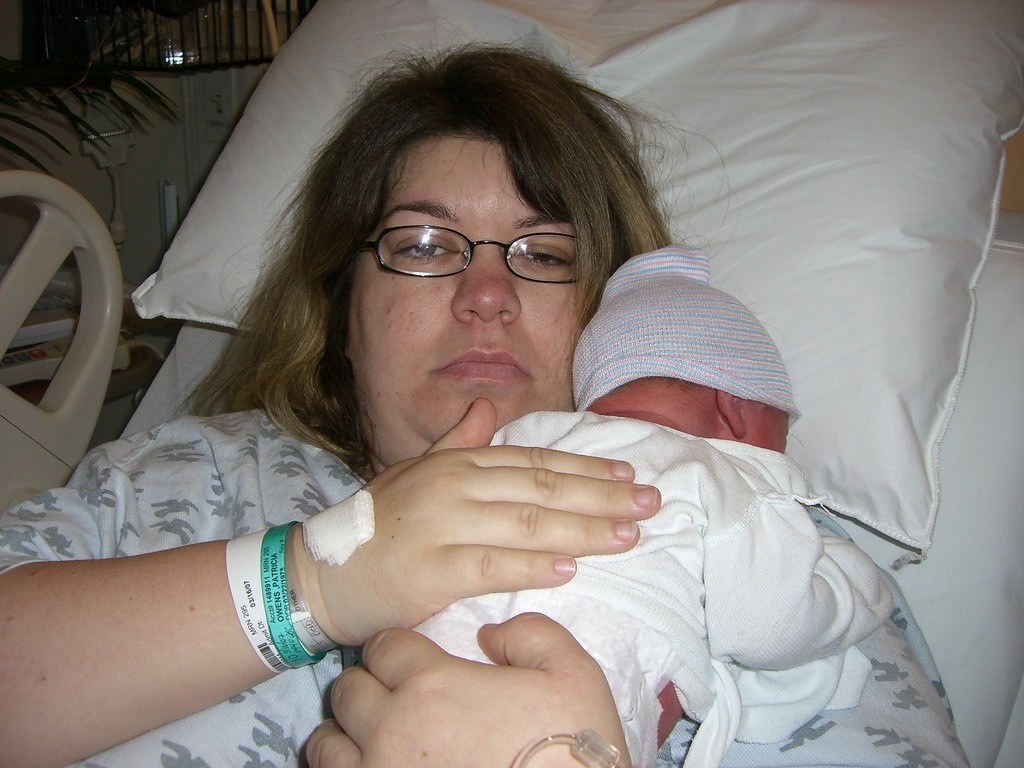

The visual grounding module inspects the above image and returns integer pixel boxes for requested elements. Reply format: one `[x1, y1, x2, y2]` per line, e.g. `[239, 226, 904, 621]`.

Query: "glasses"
[358, 225, 578, 284]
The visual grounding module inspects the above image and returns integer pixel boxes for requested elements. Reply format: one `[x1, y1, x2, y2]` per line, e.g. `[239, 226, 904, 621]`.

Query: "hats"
[573, 247, 801, 429]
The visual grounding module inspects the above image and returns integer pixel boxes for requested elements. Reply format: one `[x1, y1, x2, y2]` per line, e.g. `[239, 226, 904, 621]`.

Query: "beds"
[0, 0, 1024, 768]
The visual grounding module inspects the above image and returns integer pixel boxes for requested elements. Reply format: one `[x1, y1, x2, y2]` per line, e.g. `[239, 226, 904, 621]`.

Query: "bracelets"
[227, 520, 336, 674]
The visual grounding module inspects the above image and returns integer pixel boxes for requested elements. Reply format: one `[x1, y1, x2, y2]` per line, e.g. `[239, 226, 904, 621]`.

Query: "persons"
[0, 44, 965, 768]
[415, 245, 893, 768]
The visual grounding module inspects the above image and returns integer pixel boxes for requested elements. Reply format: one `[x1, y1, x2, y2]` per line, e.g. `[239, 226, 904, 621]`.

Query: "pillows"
[126, 0, 1024, 565]
